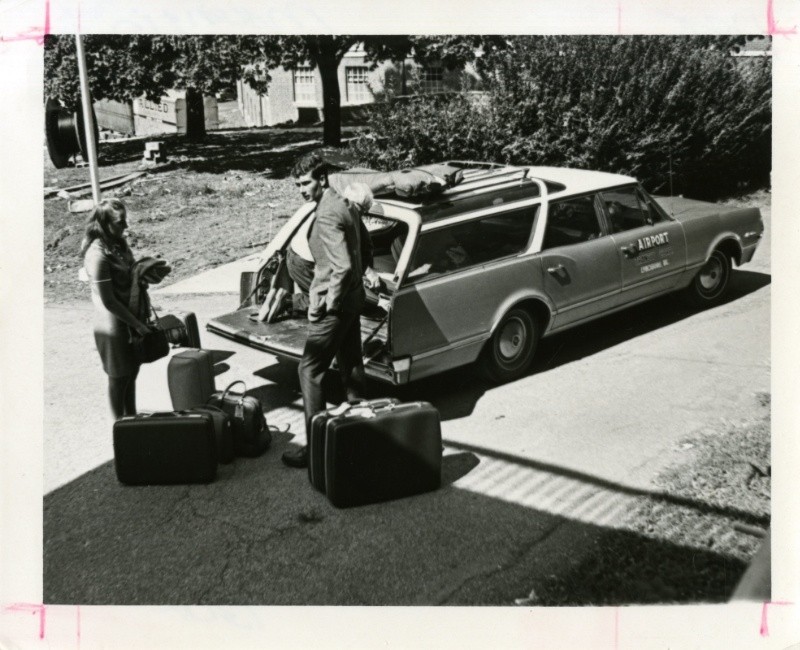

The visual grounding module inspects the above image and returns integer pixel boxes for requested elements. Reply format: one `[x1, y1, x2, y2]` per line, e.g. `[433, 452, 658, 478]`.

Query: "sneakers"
[267, 287, 289, 323]
[258, 287, 277, 321]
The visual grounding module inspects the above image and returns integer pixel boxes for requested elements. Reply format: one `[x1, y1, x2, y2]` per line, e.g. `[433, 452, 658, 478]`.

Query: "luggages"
[168, 349, 213, 412]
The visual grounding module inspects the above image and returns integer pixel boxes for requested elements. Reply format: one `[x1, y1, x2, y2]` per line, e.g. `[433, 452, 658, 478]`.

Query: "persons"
[80, 199, 169, 417]
[281, 154, 373, 467]
[257, 182, 392, 319]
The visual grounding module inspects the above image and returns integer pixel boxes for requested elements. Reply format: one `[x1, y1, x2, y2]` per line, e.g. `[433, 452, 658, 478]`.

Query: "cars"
[205, 157, 763, 387]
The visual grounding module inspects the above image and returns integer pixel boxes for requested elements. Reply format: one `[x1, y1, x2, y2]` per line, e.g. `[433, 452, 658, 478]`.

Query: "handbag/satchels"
[129, 323, 169, 363]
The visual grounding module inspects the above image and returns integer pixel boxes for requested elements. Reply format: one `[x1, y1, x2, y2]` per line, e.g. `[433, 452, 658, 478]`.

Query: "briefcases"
[181, 403, 241, 465]
[306, 396, 402, 497]
[206, 380, 272, 458]
[112, 408, 218, 486]
[321, 400, 442, 510]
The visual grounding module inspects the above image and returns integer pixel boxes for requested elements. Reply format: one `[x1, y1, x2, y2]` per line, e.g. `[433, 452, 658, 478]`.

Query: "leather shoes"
[281, 445, 310, 469]
